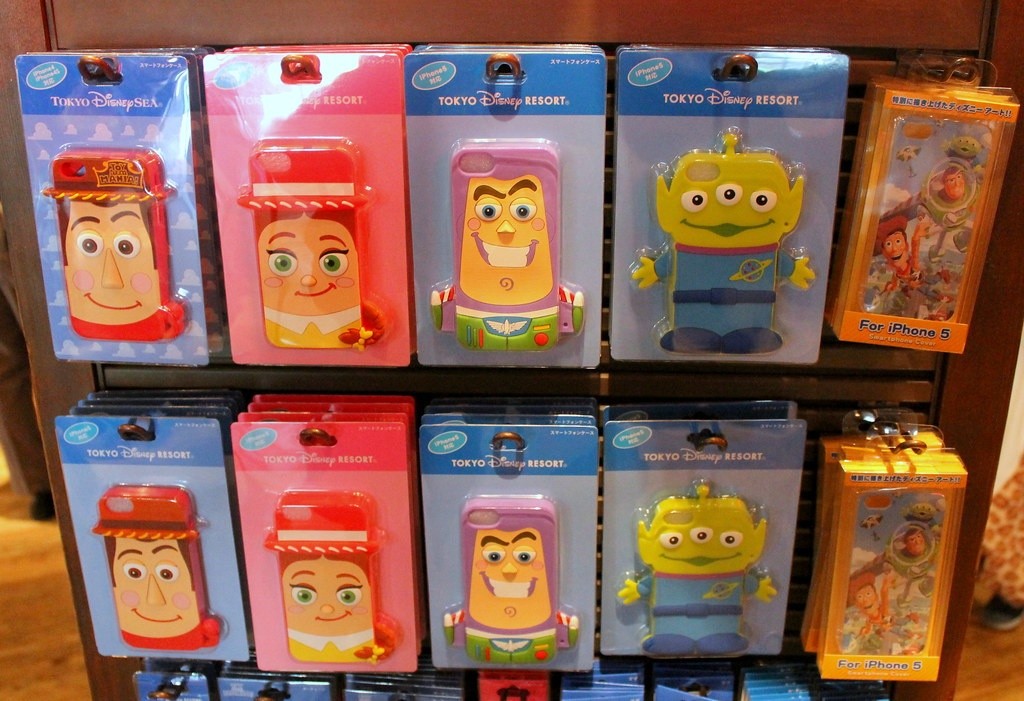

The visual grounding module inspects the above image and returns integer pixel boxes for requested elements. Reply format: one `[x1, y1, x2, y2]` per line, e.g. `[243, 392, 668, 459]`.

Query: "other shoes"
[33, 493, 55, 521]
[982, 595, 1024, 631]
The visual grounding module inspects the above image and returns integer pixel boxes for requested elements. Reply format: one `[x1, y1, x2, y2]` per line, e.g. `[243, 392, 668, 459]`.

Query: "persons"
[974, 450, 1024, 629]
